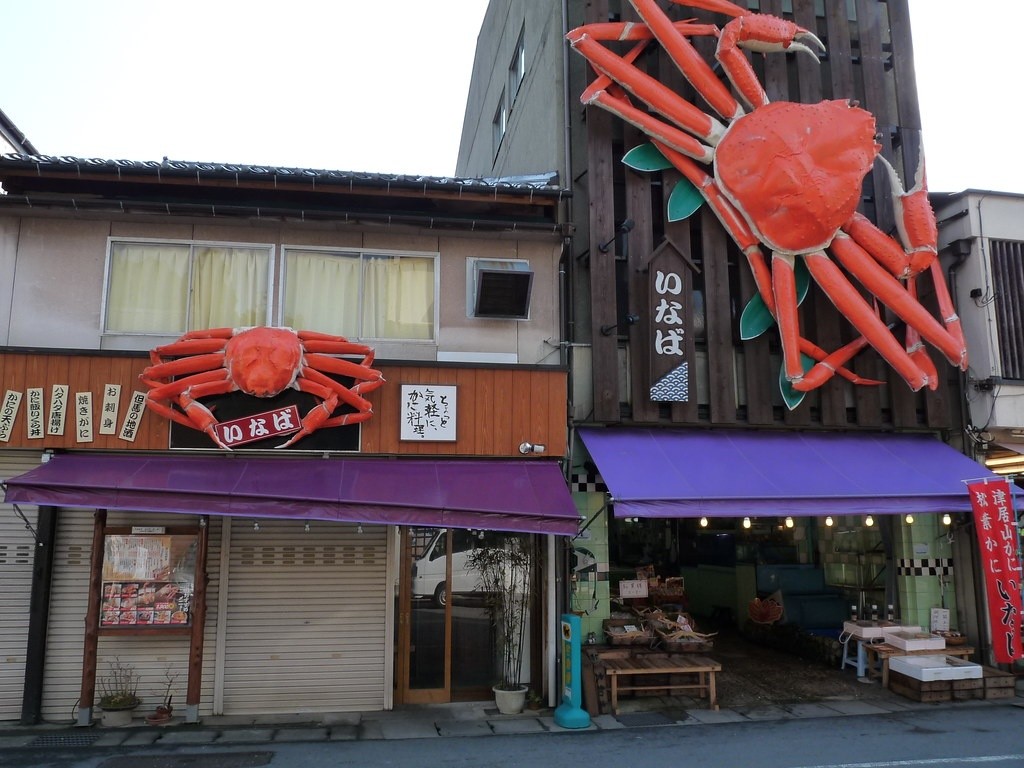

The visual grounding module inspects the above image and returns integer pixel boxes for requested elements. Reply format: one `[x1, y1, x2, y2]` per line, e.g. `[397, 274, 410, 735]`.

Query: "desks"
[599, 654, 721, 712]
[861, 642, 976, 688]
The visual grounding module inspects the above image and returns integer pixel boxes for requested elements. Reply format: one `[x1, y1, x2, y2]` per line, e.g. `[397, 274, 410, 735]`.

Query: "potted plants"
[462, 531, 548, 715]
[155, 669, 181, 718]
[96, 656, 141, 709]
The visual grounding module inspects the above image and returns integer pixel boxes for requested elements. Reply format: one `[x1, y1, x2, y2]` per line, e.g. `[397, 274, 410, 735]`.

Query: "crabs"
[563, 0, 970, 394]
[138, 325, 387, 451]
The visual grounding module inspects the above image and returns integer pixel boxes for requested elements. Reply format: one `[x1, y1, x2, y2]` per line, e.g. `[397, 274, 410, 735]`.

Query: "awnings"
[3, 454, 583, 538]
[578, 427, 1024, 518]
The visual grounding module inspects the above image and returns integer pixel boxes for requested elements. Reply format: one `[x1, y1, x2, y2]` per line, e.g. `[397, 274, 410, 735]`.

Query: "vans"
[412, 529, 530, 606]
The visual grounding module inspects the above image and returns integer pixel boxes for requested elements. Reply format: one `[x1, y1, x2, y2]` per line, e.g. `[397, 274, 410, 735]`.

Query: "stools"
[841, 636, 883, 677]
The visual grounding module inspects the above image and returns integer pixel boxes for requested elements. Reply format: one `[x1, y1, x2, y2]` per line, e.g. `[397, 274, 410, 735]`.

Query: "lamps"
[598, 218, 636, 253]
[600, 313, 641, 337]
